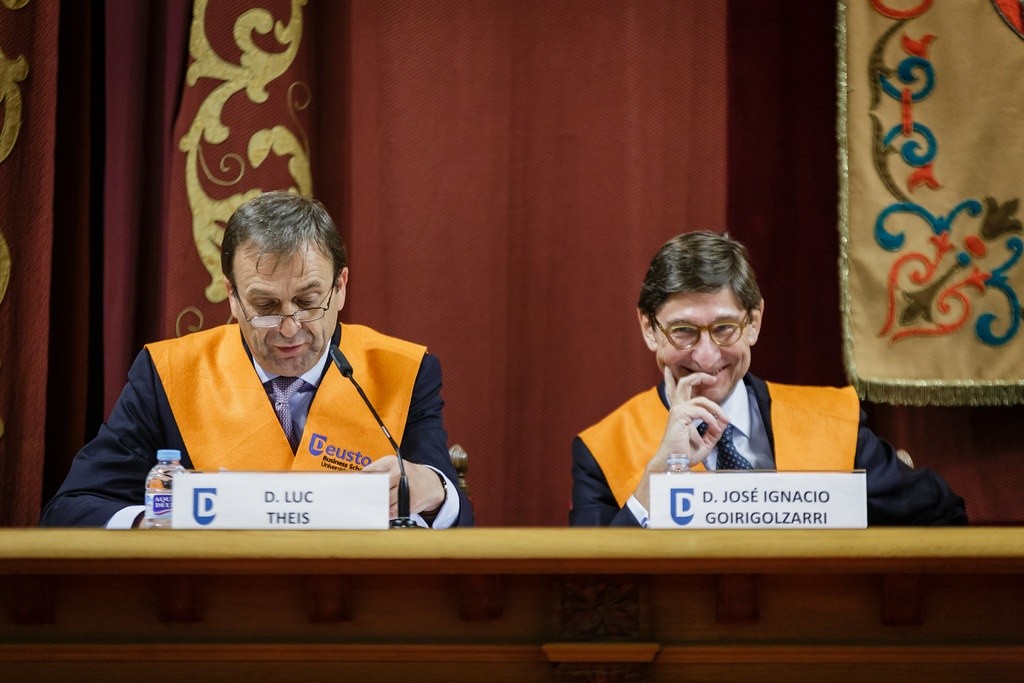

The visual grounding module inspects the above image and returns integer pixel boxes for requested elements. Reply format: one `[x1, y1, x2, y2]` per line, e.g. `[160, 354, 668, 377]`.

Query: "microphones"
[330, 347, 418, 529]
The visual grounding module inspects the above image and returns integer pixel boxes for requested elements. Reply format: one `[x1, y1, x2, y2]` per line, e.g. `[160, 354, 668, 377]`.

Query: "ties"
[695, 420, 755, 471]
[267, 375, 306, 455]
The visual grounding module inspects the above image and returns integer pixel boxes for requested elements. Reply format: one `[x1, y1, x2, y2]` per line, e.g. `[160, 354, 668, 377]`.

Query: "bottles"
[666, 454, 691, 474]
[144, 450, 186, 528]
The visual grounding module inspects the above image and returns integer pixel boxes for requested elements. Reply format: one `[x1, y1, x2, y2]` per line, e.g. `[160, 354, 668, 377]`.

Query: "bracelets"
[438, 473, 448, 493]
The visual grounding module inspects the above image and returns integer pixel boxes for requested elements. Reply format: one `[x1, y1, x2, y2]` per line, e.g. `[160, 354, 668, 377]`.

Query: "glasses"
[652, 308, 755, 350]
[230, 271, 339, 328]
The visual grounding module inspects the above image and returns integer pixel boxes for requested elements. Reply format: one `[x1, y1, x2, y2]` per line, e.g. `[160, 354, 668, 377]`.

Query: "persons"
[39, 195, 472, 530]
[571, 230, 970, 527]
